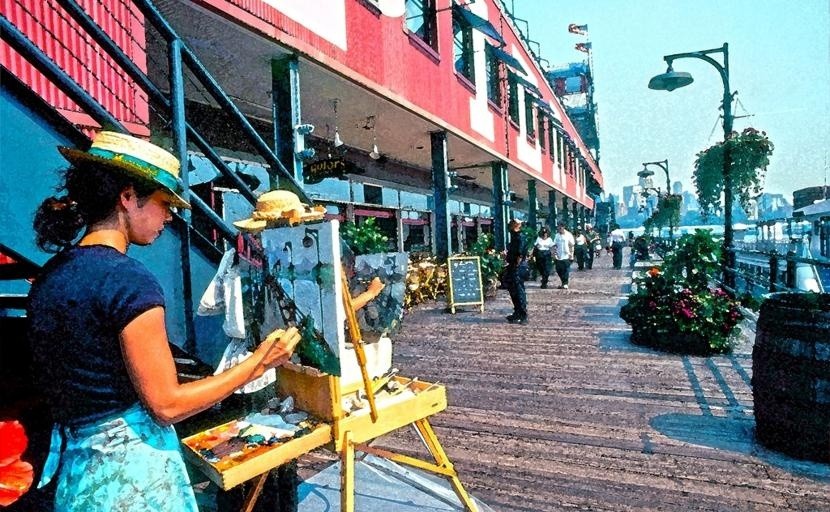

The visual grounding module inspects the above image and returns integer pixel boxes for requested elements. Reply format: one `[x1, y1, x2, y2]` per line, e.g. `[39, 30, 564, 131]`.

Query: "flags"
[568, 23, 587, 35]
[575, 41, 592, 52]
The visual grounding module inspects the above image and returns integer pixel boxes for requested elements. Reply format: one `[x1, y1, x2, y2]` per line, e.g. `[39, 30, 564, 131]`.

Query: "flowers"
[618, 228, 745, 359]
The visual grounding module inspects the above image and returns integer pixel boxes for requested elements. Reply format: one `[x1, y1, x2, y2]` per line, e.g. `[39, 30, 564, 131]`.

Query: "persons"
[404, 247, 510, 310]
[198, 189, 387, 512]
[533, 227, 555, 288]
[584, 223, 599, 269]
[609, 224, 625, 270]
[629, 231, 634, 239]
[27, 128, 303, 511]
[503, 219, 530, 324]
[822, 215, 830, 257]
[553, 223, 575, 289]
[573, 230, 588, 270]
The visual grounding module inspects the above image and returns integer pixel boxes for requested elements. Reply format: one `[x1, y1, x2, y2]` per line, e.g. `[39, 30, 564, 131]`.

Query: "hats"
[56, 129, 197, 212]
[231, 189, 326, 235]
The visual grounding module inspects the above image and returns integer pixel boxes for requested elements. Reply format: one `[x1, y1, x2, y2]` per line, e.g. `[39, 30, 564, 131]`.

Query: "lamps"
[332, 96, 382, 161]
